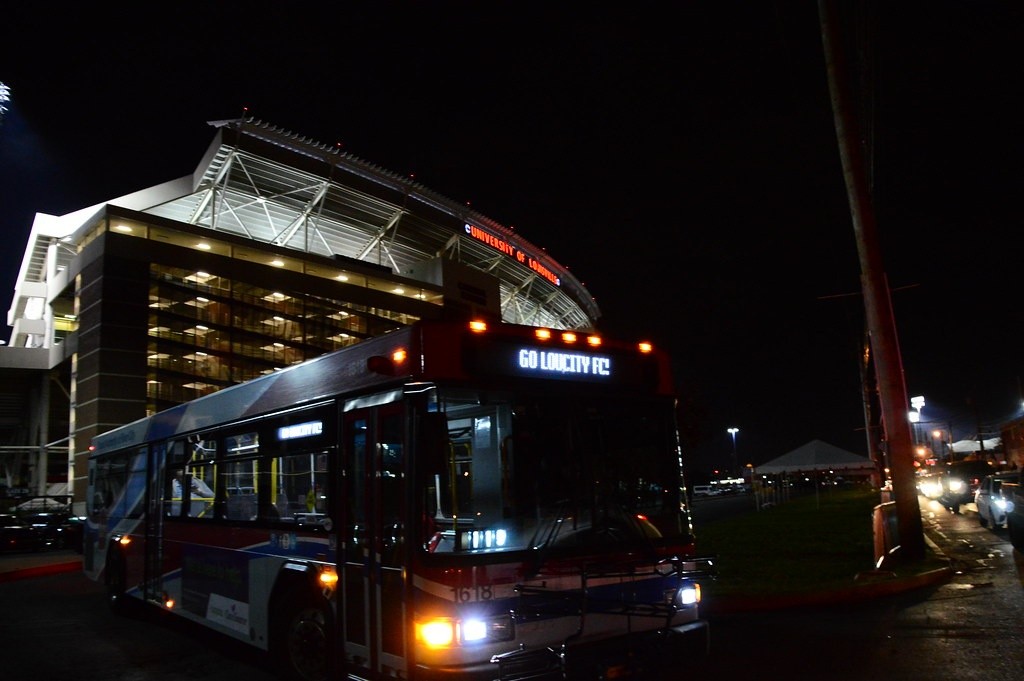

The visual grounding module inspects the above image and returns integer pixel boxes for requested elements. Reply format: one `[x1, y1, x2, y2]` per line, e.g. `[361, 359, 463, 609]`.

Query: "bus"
[82, 318, 718, 681]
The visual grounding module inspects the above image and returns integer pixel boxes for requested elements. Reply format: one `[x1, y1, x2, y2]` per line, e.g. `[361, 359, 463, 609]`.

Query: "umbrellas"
[751, 439, 875, 513]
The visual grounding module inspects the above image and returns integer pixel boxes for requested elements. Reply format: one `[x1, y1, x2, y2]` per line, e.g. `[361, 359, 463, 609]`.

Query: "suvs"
[693, 485, 721, 498]
[942, 460, 995, 512]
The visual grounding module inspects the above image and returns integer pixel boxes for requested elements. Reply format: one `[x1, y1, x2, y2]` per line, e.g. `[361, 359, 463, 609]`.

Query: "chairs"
[225, 487, 289, 519]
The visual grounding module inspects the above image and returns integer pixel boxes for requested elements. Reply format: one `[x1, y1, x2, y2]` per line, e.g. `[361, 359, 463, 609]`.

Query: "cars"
[915, 473, 943, 496]
[975, 471, 1024, 530]
